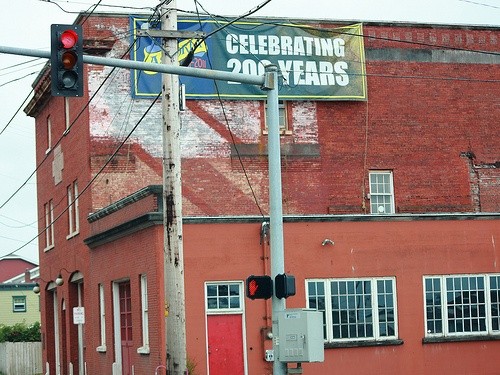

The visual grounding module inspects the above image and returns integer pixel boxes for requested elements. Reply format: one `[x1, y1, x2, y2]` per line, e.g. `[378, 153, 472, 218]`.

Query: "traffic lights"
[50, 24, 84, 97]
[246, 275, 273, 300]
[275, 273, 296, 299]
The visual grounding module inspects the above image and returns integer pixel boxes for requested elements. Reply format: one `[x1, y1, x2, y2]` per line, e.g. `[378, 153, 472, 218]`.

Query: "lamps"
[55, 267, 72, 286]
[32, 277, 47, 294]
